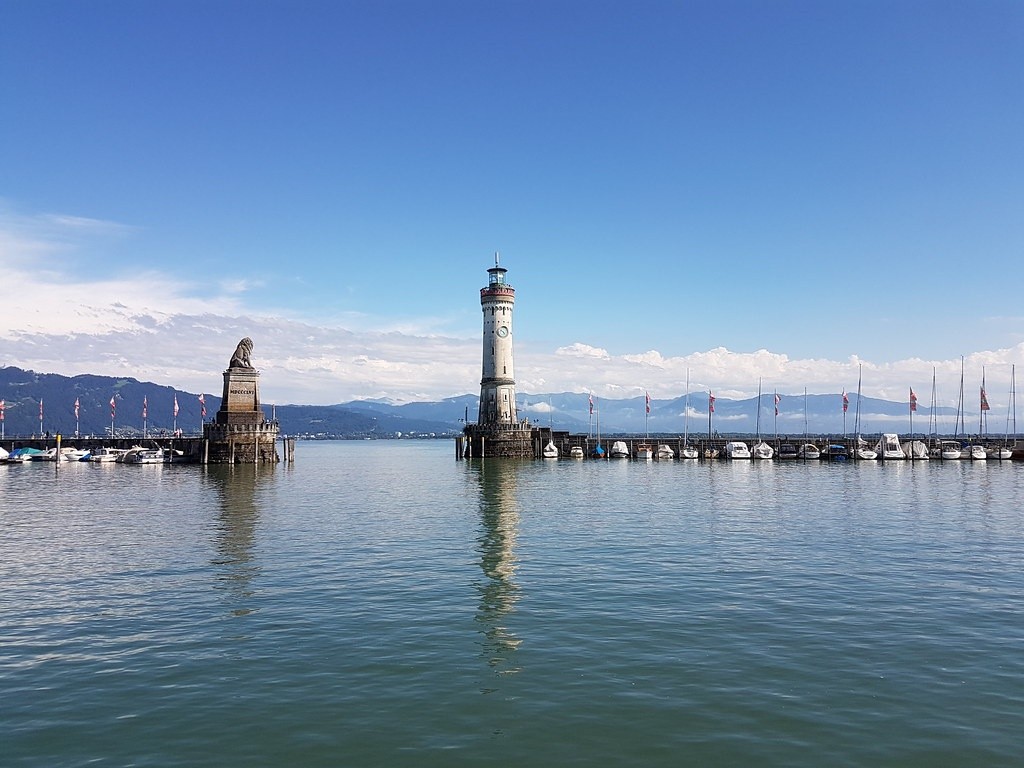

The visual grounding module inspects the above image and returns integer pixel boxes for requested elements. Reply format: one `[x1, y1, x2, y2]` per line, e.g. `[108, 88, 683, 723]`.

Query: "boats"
[901, 440, 930, 461]
[654, 444, 675, 459]
[961, 445, 987, 460]
[723, 442, 752, 459]
[571, 446, 584, 459]
[637, 444, 653, 460]
[705, 449, 721, 458]
[821, 443, 849, 462]
[611, 441, 630, 458]
[680, 402, 699, 459]
[0, 446, 189, 463]
[780, 442, 797, 459]
[798, 444, 821, 459]
[749, 438, 774, 459]
[872, 433, 906, 460]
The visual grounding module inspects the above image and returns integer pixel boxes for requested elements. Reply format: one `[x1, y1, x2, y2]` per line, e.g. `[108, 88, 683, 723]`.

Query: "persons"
[41, 431, 52, 439]
[175, 427, 183, 438]
[160, 428, 166, 437]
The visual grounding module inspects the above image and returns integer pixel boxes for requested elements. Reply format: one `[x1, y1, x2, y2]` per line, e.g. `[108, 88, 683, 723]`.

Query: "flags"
[142, 398, 147, 417]
[981, 390, 989, 410]
[110, 396, 116, 418]
[842, 391, 848, 412]
[39, 400, 43, 420]
[174, 397, 179, 416]
[199, 394, 206, 416]
[646, 393, 651, 414]
[0, 400, 5, 420]
[75, 400, 79, 416]
[775, 394, 781, 415]
[590, 398, 593, 414]
[910, 388, 917, 411]
[710, 393, 716, 412]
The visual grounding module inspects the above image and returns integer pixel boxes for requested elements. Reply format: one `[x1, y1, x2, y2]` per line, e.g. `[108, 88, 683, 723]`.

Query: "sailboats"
[849, 364, 879, 461]
[924, 356, 963, 460]
[592, 396, 605, 458]
[984, 364, 1017, 459]
[544, 396, 560, 458]
